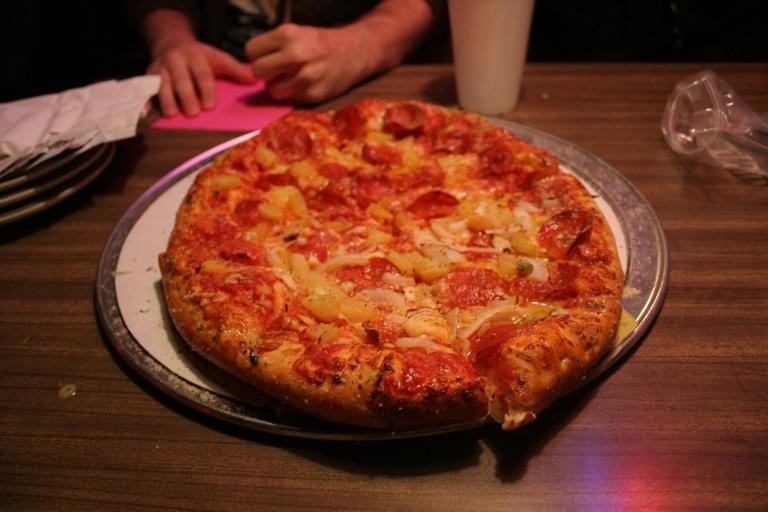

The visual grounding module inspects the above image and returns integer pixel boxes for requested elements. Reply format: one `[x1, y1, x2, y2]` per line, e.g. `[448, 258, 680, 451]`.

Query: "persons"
[129, 1, 434, 118]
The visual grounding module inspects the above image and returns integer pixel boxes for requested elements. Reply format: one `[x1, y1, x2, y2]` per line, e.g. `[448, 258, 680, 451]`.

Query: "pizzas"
[158, 98, 624, 434]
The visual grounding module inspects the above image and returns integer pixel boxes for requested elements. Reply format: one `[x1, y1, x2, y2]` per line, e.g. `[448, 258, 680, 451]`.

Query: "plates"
[1, 139, 123, 235]
[90, 107, 667, 446]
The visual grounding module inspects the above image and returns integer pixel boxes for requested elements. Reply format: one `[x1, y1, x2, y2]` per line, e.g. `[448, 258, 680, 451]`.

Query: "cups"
[447, 1, 533, 114]
